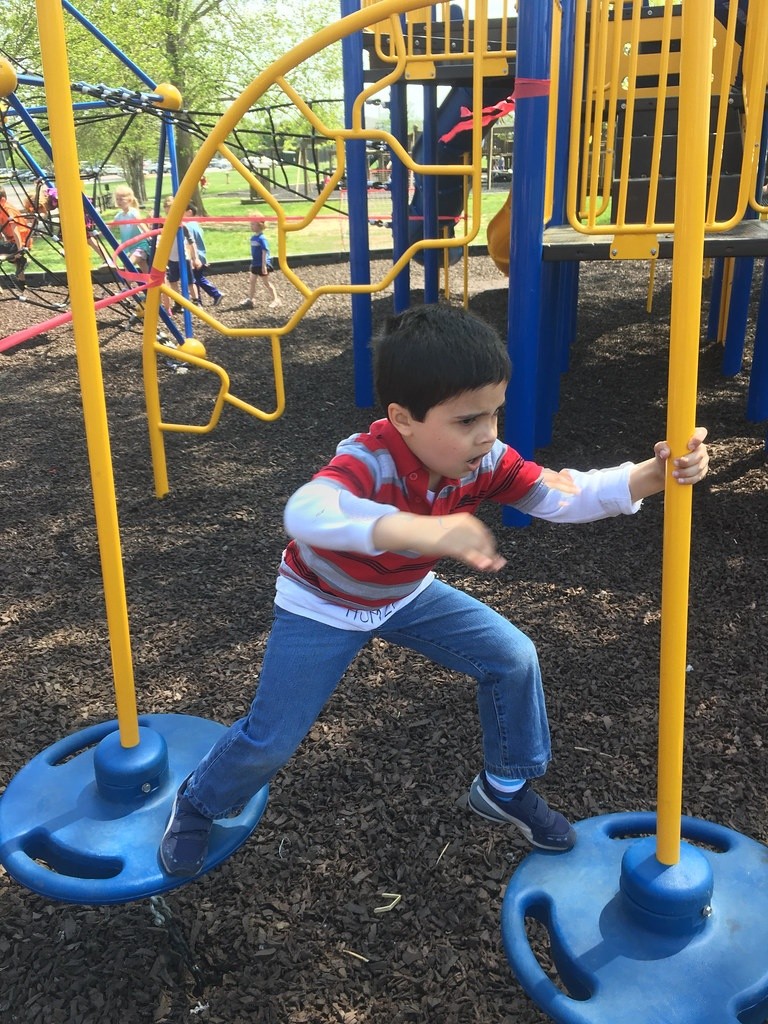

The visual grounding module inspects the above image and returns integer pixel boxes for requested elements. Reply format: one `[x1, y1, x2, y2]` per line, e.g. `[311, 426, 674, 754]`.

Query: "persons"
[145, 196, 201, 319]
[158, 304, 708, 877]
[387, 149, 411, 214]
[0, 186, 32, 301]
[492, 153, 505, 179]
[182, 200, 225, 305]
[100, 186, 152, 293]
[200, 176, 208, 195]
[240, 213, 280, 308]
[24, 188, 110, 274]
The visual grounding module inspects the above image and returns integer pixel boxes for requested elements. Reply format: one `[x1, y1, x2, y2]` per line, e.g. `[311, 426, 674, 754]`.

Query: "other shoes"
[213, 290, 225, 306]
[170, 306, 184, 312]
[166, 307, 173, 317]
[57, 231, 62, 241]
[98, 263, 118, 275]
[15, 257, 28, 277]
[269, 298, 283, 307]
[6, 279, 26, 291]
[121, 286, 133, 291]
[191, 301, 203, 310]
[240, 298, 255, 307]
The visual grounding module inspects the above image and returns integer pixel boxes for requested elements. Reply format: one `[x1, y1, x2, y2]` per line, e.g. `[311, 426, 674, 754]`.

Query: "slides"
[485, 189, 510, 278]
[408, 84, 515, 275]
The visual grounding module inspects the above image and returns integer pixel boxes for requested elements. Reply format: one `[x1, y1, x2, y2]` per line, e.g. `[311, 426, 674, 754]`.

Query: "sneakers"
[159, 770, 214, 878]
[467, 770, 578, 852]
